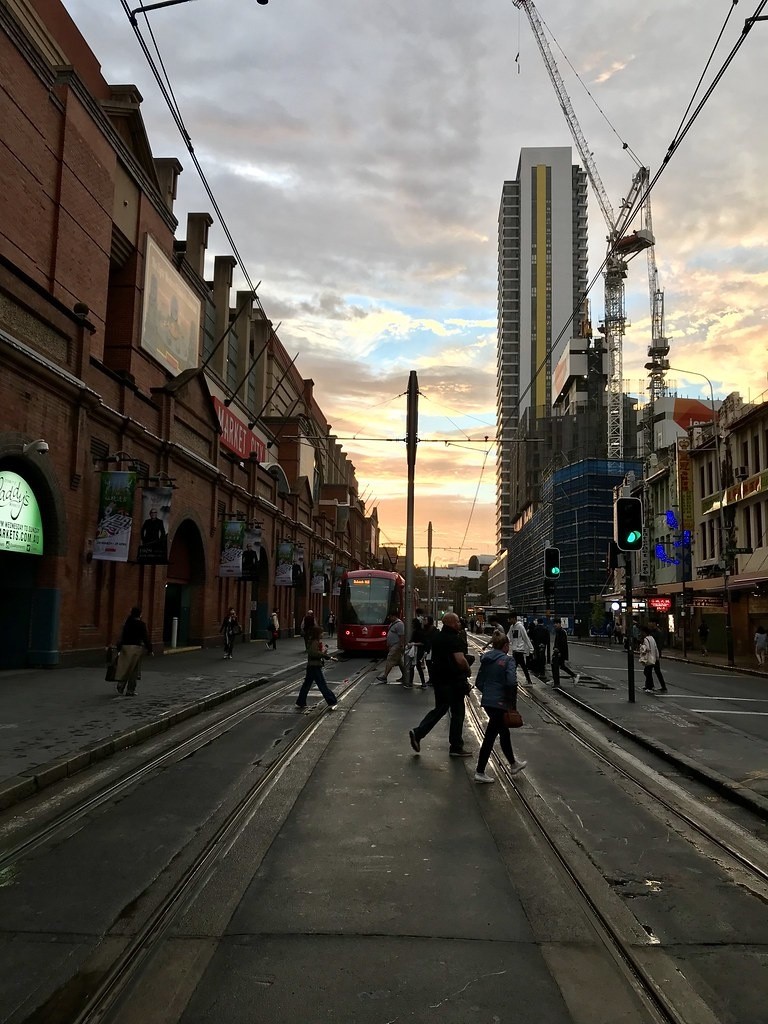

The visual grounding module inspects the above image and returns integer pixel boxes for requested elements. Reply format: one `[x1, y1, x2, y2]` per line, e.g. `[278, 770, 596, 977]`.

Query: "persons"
[296, 626, 337, 707]
[403, 608, 440, 690]
[697, 619, 710, 656]
[754, 626, 768, 666]
[376, 610, 405, 683]
[459, 617, 481, 634]
[265, 608, 279, 650]
[410, 612, 473, 757]
[114, 606, 154, 696]
[242, 543, 258, 564]
[473, 629, 527, 782]
[326, 610, 335, 638]
[506, 612, 534, 686]
[141, 508, 166, 546]
[640, 626, 668, 693]
[219, 607, 238, 659]
[482, 615, 504, 651]
[293, 560, 301, 576]
[606, 621, 622, 645]
[301, 610, 317, 648]
[551, 619, 581, 686]
[527, 618, 549, 679]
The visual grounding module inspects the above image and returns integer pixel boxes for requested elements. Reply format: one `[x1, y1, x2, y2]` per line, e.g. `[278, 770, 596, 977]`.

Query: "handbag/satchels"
[638, 652, 648, 665]
[503, 707, 523, 728]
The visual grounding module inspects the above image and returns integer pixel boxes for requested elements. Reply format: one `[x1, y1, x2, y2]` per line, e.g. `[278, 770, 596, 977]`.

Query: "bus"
[336, 570, 420, 651]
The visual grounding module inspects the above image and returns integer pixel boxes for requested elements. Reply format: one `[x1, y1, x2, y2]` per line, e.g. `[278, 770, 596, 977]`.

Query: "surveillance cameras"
[36, 441, 49, 455]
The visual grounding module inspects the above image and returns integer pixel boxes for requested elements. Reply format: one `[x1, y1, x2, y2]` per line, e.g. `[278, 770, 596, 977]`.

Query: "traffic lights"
[615, 496, 644, 552]
[544, 547, 560, 579]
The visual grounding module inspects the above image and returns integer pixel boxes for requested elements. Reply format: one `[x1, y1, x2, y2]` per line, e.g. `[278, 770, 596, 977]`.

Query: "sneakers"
[409, 728, 420, 752]
[545, 679, 554, 685]
[573, 674, 580, 686]
[449, 746, 473, 758]
[511, 760, 527, 775]
[474, 772, 495, 784]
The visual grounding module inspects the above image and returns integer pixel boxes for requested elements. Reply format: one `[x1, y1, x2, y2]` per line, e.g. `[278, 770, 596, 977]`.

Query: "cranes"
[512, 0, 668, 476]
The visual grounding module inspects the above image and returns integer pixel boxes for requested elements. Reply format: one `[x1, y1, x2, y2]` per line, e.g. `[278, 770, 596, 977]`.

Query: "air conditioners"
[734, 466, 749, 477]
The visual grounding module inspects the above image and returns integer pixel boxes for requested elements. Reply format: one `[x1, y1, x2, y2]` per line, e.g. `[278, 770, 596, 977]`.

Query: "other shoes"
[642, 686, 667, 694]
[425, 681, 433, 686]
[223, 655, 232, 660]
[295, 703, 308, 709]
[533, 670, 548, 679]
[376, 675, 387, 683]
[401, 684, 413, 689]
[523, 682, 533, 688]
[117, 685, 124, 695]
[329, 703, 338, 710]
[758, 662, 765, 670]
[273, 645, 276, 650]
[266, 642, 272, 652]
[396, 678, 404, 682]
[419, 685, 427, 690]
[125, 692, 139, 697]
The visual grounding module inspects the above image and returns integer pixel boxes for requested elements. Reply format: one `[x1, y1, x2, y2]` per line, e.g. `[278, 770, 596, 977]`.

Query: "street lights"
[546, 500, 581, 618]
[644, 363, 735, 666]
